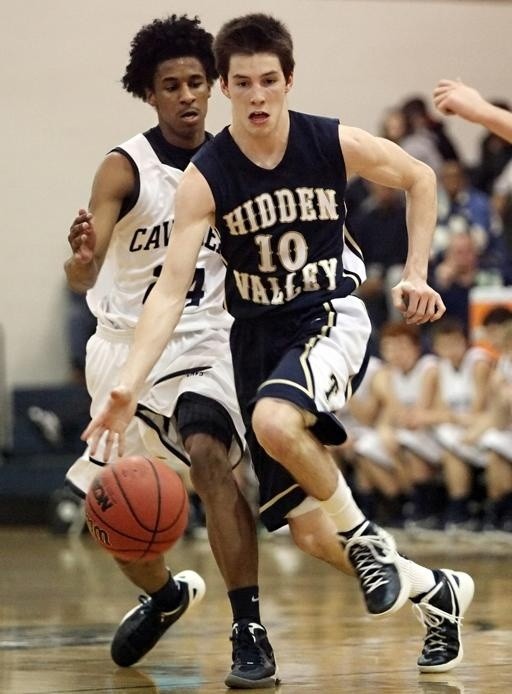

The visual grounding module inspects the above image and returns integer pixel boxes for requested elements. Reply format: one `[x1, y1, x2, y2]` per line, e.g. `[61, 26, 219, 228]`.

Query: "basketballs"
[84, 452, 189, 561]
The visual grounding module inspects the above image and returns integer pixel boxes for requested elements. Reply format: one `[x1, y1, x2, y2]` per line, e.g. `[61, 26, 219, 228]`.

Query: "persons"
[430, 75, 511, 149]
[322, 89, 511, 548]
[79, 11, 481, 677]
[53, 14, 285, 688]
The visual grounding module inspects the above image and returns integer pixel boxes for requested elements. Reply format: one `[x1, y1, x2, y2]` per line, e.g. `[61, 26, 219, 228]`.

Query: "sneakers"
[226, 621, 281, 687]
[337, 521, 408, 619]
[111, 570, 206, 667]
[409, 569, 476, 674]
